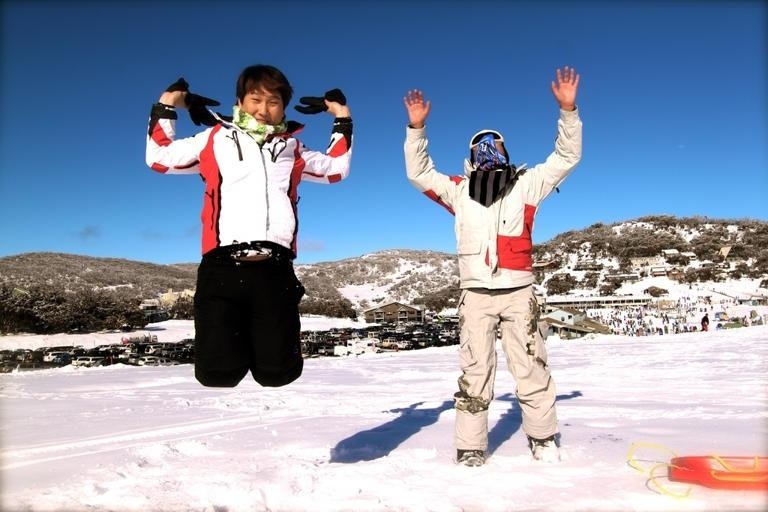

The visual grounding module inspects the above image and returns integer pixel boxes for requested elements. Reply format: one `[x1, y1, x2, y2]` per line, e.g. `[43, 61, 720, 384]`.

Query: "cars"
[497, 326, 502, 339]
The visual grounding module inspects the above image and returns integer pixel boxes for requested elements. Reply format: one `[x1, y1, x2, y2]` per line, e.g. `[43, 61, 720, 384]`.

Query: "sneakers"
[455, 448, 488, 469]
[529, 436, 558, 462]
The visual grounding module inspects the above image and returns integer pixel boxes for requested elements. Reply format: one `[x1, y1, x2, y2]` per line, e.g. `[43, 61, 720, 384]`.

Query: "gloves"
[168, 79, 221, 125]
[295, 88, 347, 115]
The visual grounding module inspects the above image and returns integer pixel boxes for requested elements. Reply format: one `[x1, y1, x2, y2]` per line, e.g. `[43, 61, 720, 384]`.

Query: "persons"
[401, 63, 584, 467]
[590, 294, 741, 337]
[144, 63, 357, 389]
